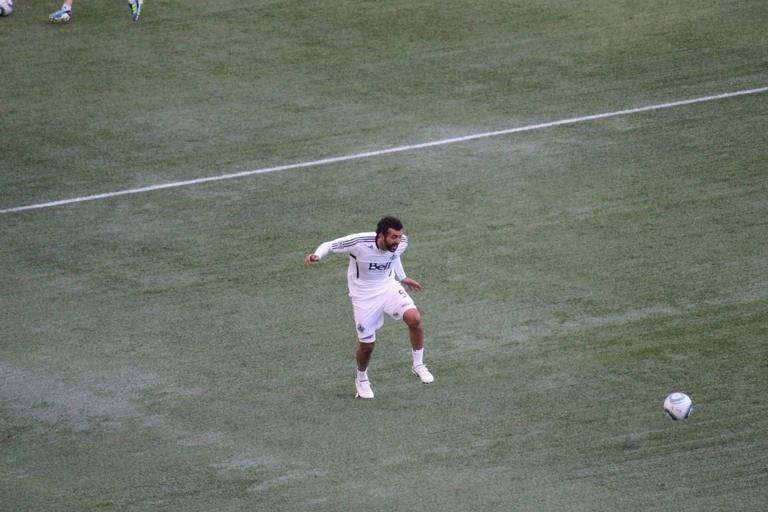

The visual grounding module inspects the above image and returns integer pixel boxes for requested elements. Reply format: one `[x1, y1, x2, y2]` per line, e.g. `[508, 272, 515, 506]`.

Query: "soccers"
[663, 393, 693, 421]
[1, 1, 14, 17]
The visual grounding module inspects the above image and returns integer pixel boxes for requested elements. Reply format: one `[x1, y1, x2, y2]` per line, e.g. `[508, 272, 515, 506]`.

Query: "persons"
[47, 1, 144, 25]
[303, 215, 435, 400]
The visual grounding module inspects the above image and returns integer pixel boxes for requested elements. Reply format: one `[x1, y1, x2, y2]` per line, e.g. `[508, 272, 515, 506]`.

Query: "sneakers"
[128, 1, 142, 22]
[354, 378, 375, 398]
[411, 362, 434, 384]
[48, 10, 71, 22]
